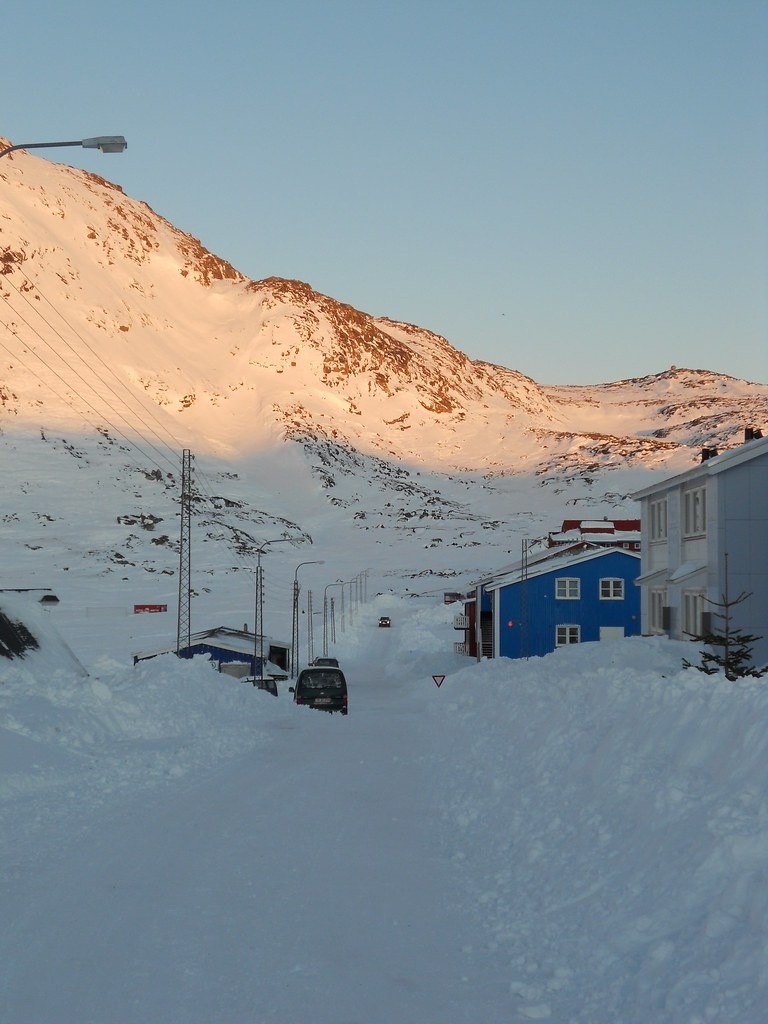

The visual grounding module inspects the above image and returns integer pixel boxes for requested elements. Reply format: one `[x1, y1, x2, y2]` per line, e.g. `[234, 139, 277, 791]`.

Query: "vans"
[289, 666, 348, 715]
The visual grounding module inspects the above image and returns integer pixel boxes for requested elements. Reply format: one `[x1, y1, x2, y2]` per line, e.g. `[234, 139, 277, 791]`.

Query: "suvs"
[309, 657, 340, 669]
[378, 617, 391, 628]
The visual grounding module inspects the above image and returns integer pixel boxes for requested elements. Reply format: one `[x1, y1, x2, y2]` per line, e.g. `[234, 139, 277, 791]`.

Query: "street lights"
[291, 559, 325, 679]
[253, 538, 307, 688]
[324, 569, 374, 656]
